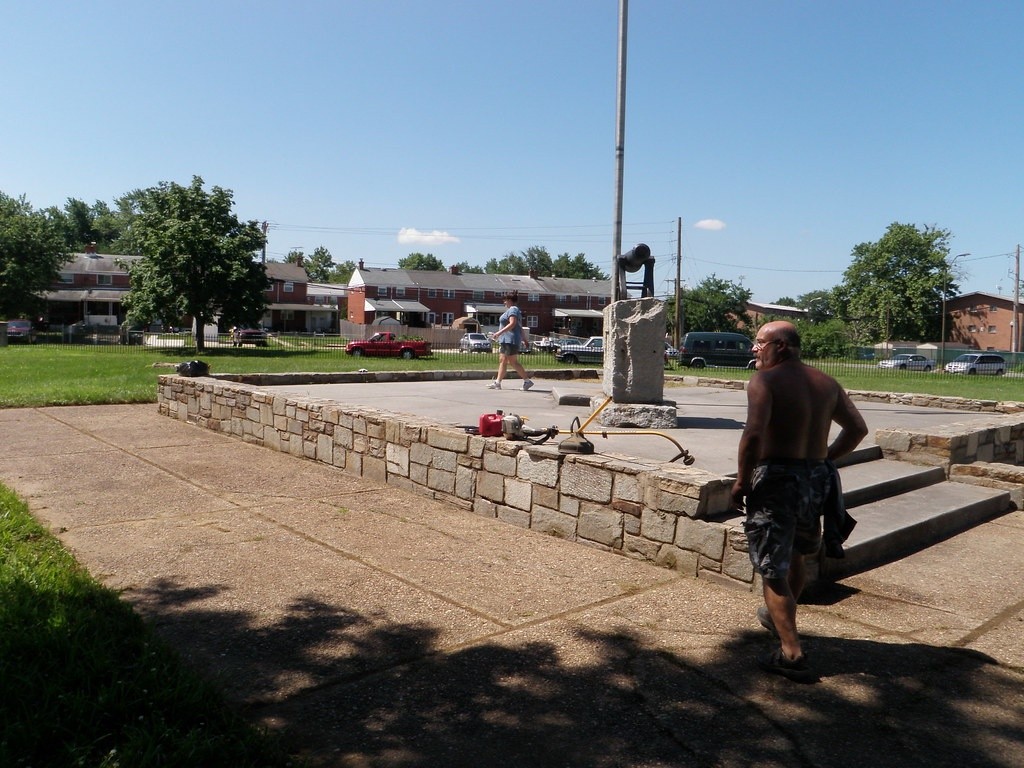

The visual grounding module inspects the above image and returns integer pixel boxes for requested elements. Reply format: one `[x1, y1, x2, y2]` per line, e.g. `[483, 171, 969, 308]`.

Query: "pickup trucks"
[344, 332, 432, 360]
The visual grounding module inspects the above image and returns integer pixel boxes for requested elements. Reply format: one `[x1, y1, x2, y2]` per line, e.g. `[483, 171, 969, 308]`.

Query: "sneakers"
[519, 380, 534, 391]
[763, 649, 815, 682]
[757, 606, 779, 639]
[486, 382, 501, 390]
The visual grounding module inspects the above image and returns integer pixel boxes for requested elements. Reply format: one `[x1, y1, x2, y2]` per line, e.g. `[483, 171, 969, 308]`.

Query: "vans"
[677, 332, 757, 371]
[943, 353, 1006, 376]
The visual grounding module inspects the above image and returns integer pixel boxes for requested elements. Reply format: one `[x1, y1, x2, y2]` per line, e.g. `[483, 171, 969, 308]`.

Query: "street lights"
[940, 253, 971, 373]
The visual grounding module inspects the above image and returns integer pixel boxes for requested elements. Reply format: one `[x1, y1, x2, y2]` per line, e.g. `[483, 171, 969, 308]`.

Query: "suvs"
[5, 319, 37, 345]
[232, 322, 270, 348]
[459, 333, 493, 353]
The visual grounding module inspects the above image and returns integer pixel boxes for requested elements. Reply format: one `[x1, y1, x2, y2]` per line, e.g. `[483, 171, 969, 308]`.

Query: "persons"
[485, 289, 534, 391]
[730, 321, 869, 680]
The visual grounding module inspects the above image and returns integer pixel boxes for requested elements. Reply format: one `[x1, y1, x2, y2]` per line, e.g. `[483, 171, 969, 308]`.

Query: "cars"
[664, 342, 679, 360]
[498, 339, 583, 355]
[554, 336, 669, 371]
[876, 354, 935, 372]
